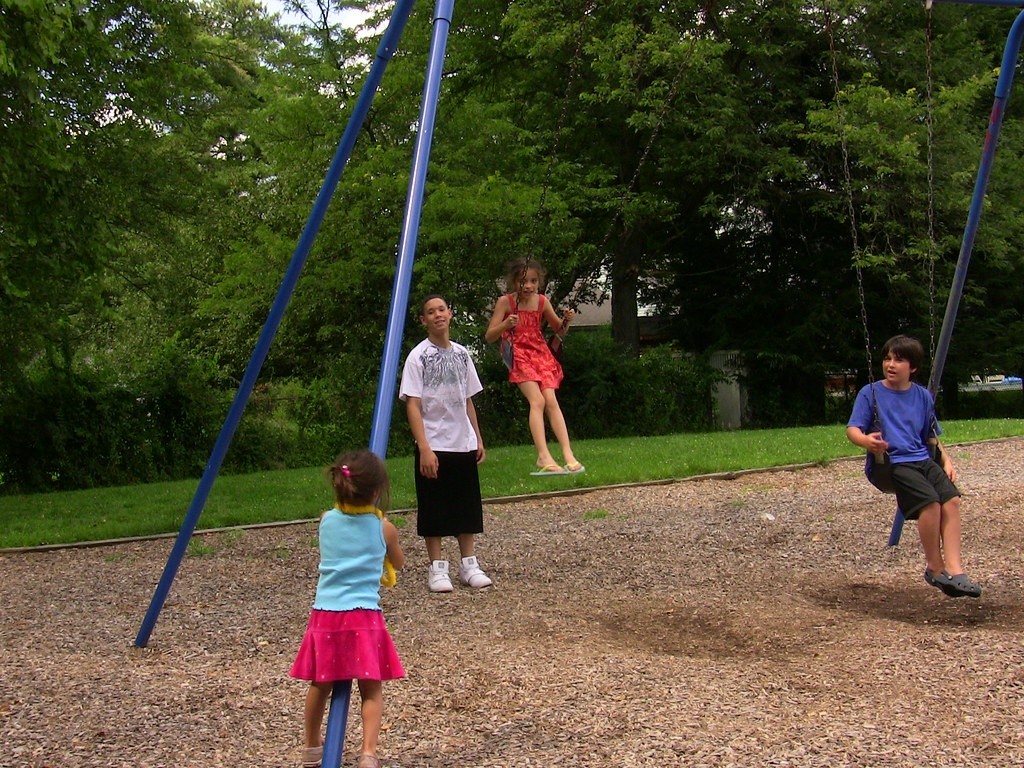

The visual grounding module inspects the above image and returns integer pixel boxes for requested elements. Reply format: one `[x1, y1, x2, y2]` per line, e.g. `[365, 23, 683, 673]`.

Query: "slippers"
[563, 461, 586, 473]
[529, 464, 568, 475]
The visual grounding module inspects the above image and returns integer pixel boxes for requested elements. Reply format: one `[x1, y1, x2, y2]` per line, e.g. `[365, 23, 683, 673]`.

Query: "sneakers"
[459, 556, 492, 588]
[428, 560, 454, 592]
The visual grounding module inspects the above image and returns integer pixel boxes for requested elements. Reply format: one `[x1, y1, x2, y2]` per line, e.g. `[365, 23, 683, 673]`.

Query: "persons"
[485, 256, 585, 473]
[288, 447, 405, 768]
[846, 334, 982, 598]
[399, 294, 494, 593]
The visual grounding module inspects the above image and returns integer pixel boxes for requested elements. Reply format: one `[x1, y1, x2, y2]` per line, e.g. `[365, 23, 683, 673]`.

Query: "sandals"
[924, 566, 964, 597]
[935, 570, 981, 598]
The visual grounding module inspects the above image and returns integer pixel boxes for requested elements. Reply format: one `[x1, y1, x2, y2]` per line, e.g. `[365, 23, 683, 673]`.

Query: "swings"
[501, 0, 717, 372]
[822, 0, 943, 495]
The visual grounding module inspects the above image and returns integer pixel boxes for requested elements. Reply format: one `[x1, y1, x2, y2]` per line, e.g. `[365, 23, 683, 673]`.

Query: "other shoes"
[300, 736, 324, 767]
[358, 754, 381, 768]
